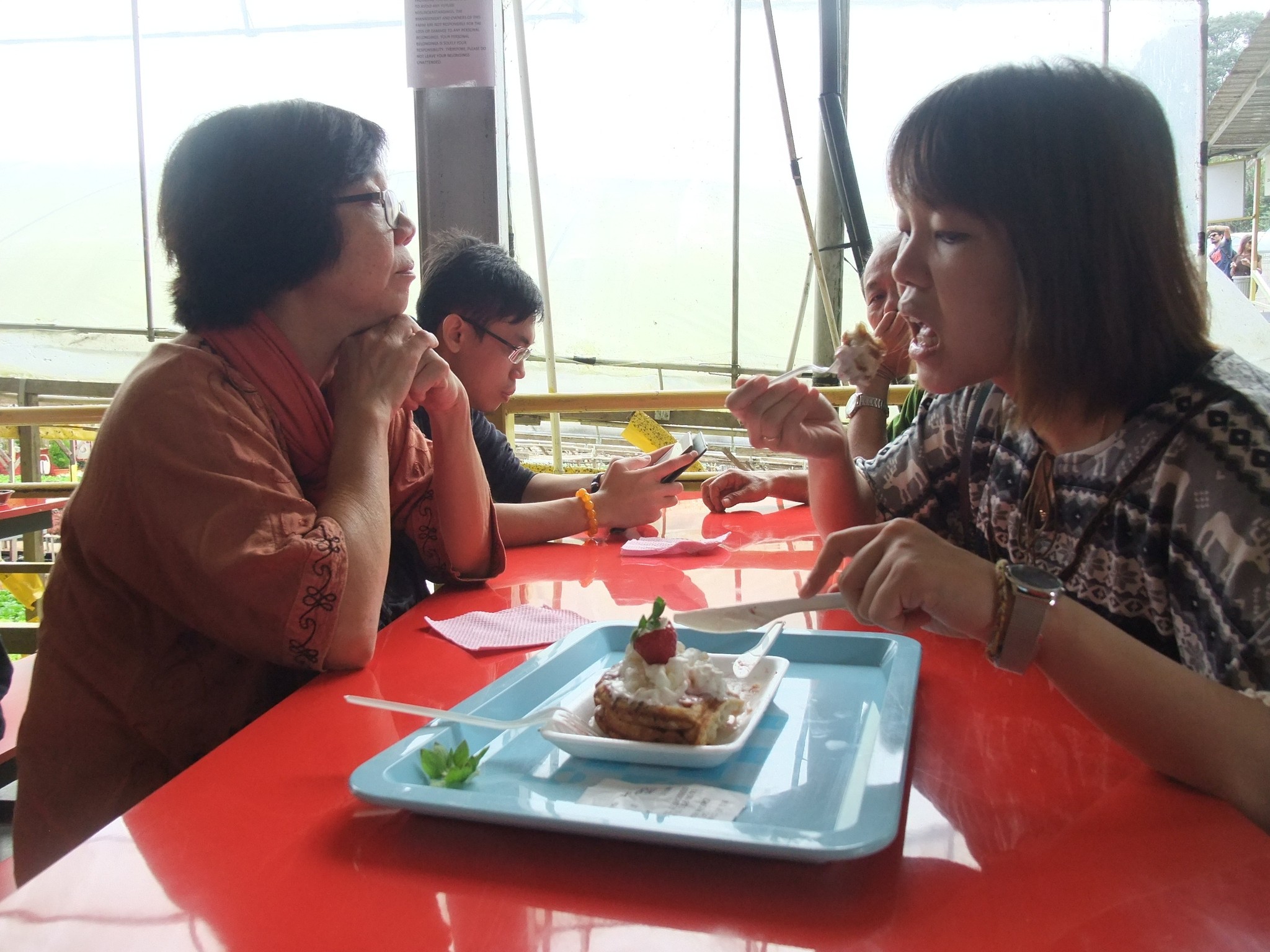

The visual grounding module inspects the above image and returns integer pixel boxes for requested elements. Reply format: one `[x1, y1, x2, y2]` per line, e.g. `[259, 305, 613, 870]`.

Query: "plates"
[538, 653, 790, 770]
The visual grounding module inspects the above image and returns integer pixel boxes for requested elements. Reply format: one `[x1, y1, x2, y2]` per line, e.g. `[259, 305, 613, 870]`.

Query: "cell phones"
[651, 432, 694, 467]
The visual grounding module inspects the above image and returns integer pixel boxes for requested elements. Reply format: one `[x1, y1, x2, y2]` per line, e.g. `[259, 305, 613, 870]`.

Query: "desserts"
[833, 323, 886, 394]
[592, 594, 746, 746]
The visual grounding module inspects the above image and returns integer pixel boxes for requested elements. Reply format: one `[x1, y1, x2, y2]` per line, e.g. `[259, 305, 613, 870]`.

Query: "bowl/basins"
[0, 489, 15, 504]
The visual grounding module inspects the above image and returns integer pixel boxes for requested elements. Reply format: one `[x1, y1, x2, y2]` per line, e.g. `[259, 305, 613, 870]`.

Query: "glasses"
[333, 190, 409, 230]
[457, 313, 532, 363]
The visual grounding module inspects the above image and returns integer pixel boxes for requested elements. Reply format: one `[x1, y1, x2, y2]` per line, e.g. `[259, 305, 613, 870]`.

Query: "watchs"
[990, 565, 1064, 675]
[844, 391, 890, 420]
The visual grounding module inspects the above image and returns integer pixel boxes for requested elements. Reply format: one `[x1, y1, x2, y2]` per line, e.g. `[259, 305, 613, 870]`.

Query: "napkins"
[620, 531, 733, 555]
[423, 603, 597, 650]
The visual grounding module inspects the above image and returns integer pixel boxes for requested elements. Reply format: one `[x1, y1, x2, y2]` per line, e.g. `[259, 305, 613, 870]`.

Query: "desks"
[0, 490, 1269, 952]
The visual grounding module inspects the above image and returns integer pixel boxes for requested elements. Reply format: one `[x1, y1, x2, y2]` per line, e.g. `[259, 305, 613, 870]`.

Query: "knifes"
[674, 592, 847, 633]
[732, 619, 787, 678]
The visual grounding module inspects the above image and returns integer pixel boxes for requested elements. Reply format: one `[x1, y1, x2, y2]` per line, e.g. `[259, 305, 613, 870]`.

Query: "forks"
[768, 358, 841, 388]
[344, 695, 604, 737]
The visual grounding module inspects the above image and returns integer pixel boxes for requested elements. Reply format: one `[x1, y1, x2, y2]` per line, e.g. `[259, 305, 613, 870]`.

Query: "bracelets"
[1212, 225, 1217, 231]
[574, 487, 598, 538]
[591, 472, 605, 493]
[986, 558, 1006, 656]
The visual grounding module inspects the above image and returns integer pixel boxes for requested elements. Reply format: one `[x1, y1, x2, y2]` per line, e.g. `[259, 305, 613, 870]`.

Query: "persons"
[12, 99, 506, 887]
[701, 231, 937, 515]
[401, 228, 696, 547]
[1206, 225, 1232, 281]
[728, 54, 1270, 835]
[1229, 235, 1262, 293]
